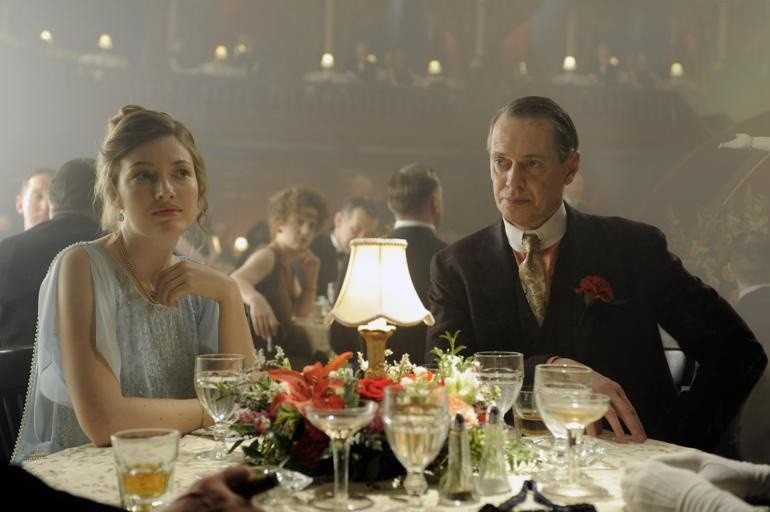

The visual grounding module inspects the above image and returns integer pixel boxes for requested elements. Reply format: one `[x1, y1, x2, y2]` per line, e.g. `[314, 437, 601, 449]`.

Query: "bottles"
[479, 408, 511, 495]
[444, 413, 480, 506]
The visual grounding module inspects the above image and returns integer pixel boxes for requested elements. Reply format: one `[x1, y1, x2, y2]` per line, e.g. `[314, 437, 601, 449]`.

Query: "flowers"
[573, 274, 615, 327]
[213, 344, 508, 468]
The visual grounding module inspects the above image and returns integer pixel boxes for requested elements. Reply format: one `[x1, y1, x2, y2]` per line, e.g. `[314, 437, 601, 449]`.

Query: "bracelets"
[200, 402, 210, 427]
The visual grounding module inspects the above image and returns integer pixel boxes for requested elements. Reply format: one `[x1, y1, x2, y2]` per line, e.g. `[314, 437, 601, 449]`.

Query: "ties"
[518, 233, 550, 328]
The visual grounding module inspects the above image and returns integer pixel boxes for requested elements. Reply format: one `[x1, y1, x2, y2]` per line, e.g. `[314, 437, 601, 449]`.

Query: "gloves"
[620, 461, 769, 512]
[649, 450, 770, 502]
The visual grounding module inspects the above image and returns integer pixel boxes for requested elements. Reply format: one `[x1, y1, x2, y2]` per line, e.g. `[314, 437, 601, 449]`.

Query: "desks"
[20, 417, 702, 512]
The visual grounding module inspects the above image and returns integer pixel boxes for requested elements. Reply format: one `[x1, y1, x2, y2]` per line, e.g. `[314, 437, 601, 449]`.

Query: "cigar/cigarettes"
[234, 472, 283, 497]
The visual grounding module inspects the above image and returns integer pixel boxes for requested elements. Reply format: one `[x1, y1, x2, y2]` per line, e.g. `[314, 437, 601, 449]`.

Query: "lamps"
[324, 238, 435, 378]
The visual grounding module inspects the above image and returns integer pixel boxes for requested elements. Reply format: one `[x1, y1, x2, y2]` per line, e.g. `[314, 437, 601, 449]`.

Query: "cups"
[109, 428, 179, 512]
[475, 352, 525, 414]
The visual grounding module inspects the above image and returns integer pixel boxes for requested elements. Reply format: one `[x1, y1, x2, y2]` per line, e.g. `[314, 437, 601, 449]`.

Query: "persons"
[1, 158, 114, 457]
[16, 169, 59, 232]
[1, 463, 269, 512]
[727, 232, 769, 464]
[4, 103, 258, 511]
[562, 167, 593, 214]
[183, 162, 454, 367]
[426, 94, 770, 450]
[620, 447, 770, 512]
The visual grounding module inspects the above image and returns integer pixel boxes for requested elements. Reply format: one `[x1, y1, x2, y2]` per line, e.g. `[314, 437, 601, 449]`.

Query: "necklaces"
[114, 231, 162, 306]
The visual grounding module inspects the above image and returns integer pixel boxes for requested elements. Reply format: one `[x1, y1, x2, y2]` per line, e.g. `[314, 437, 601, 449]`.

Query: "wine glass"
[541, 393, 610, 498]
[193, 353, 250, 463]
[533, 363, 594, 466]
[514, 390, 551, 473]
[382, 385, 451, 511]
[303, 401, 379, 511]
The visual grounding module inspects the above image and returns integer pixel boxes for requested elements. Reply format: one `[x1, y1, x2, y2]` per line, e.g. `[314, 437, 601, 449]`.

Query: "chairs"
[2, 345, 51, 510]
